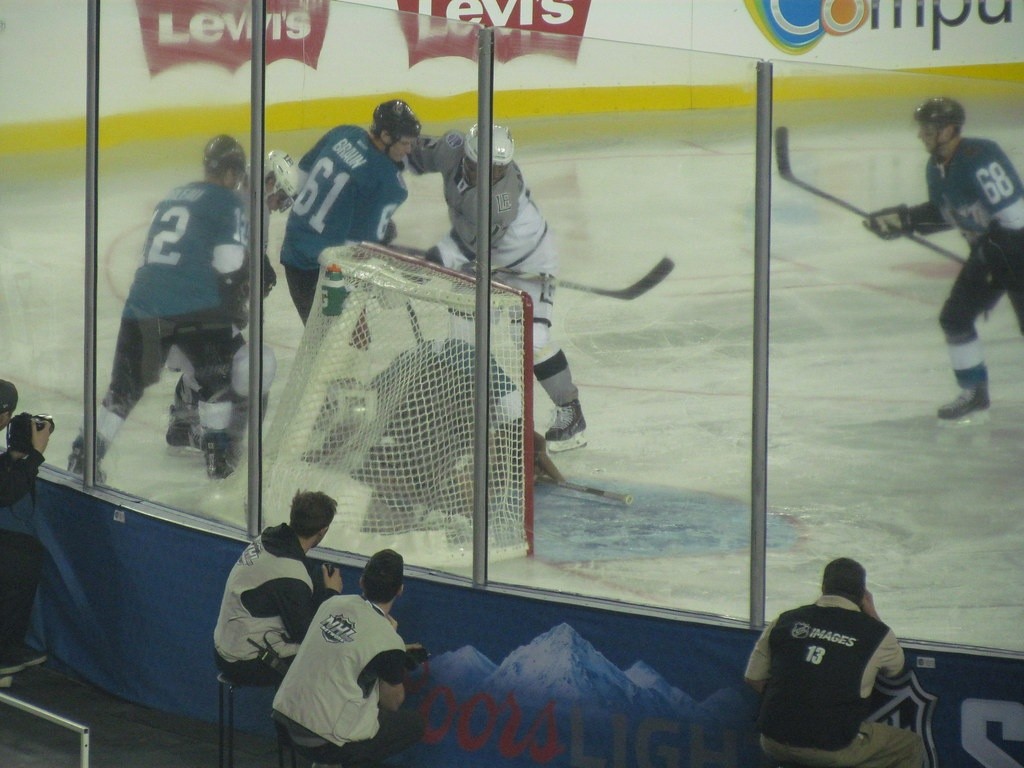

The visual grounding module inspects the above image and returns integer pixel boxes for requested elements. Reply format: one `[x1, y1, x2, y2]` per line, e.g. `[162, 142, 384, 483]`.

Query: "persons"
[0, 376, 51, 673]
[271, 547, 424, 768]
[214, 487, 345, 685]
[743, 556, 927, 768]
[863, 96, 1024, 420]
[66, 134, 299, 481]
[276, 98, 422, 349]
[402, 120, 589, 451]
[303, 337, 565, 543]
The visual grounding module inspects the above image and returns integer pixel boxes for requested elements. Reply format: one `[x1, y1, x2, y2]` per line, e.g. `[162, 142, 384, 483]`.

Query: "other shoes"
[203, 439, 242, 481]
[66, 432, 111, 486]
[164, 417, 216, 459]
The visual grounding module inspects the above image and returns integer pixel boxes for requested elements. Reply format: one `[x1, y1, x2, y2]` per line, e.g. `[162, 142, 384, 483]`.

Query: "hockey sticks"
[386, 244, 676, 301]
[538, 476, 633, 507]
[775, 126, 970, 268]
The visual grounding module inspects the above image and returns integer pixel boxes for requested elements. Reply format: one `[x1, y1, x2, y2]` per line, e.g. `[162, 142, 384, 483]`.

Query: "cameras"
[8, 412, 55, 454]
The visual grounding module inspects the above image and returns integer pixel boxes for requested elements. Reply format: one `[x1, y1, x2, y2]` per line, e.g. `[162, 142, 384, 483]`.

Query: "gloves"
[862, 201, 916, 242]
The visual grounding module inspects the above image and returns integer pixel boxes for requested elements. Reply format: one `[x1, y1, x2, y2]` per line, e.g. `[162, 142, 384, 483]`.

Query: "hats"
[1, 380, 18, 415]
[361, 549, 405, 604]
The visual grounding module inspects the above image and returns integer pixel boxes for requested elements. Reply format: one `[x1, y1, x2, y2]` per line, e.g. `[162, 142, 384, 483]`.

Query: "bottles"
[321, 264, 344, 317]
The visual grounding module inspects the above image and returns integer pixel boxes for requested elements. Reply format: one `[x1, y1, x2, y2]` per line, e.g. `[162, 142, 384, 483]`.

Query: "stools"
[217, 673, 284, 768]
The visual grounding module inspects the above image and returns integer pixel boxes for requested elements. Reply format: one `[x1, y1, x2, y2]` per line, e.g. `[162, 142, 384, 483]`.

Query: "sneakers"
[544, 402, 588, 453]
[934, 385, 993, 429]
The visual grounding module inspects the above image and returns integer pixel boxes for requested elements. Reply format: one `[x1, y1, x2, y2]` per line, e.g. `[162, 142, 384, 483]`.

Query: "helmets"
[463, 123, 515, 167]
[371, 99, 422, 138]
[261, 149, 301, 214]
[912, 96, 966, 126]
[202, 135, 249, 180]
